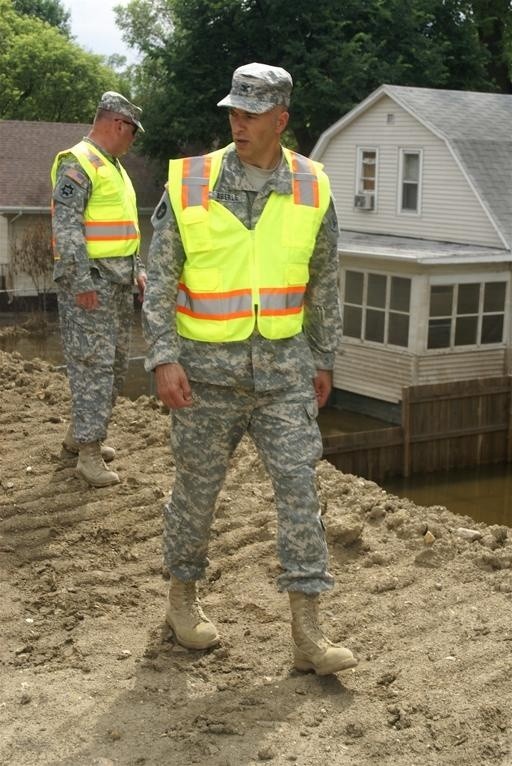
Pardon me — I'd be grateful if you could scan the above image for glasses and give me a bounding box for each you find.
[114,119,139,135]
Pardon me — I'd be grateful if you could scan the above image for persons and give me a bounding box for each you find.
[50,91,147,487]
[141,62,359,675]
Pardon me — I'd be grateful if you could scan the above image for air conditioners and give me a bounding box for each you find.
[354,194,374,210]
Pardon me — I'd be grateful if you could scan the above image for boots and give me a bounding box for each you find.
[73,439,122,488]
[164,564,222,652]
[60,422,117,463]
[287,589,359,678]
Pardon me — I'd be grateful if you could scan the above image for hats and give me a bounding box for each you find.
[96,90,145,134]
[216,62,294,115]
